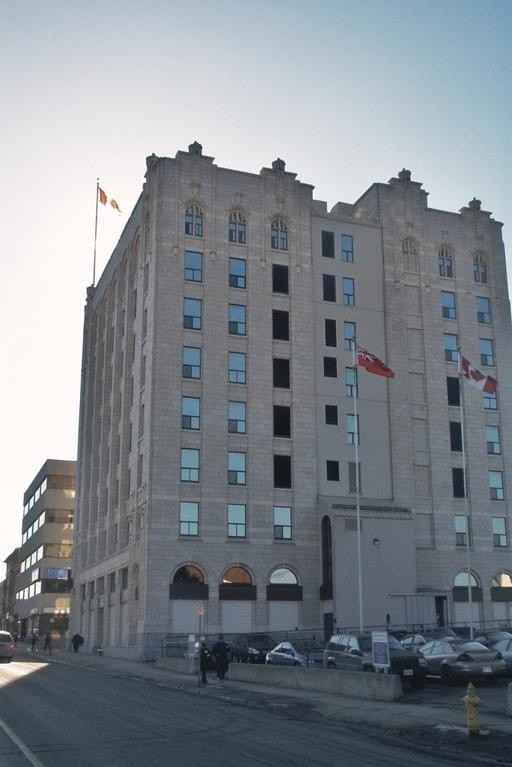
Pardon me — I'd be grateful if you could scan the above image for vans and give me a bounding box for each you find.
[0,629,15,663]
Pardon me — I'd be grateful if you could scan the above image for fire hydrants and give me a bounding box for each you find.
[462,682,483,739]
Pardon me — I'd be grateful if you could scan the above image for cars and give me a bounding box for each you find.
[403,626,512,685]
[183,633,325,668]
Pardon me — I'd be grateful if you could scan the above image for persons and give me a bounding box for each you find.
[212,634,231,681]
[10,629,85,656]
[198,641,212,687]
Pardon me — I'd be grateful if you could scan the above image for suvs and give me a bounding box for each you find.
[323,631,427,690]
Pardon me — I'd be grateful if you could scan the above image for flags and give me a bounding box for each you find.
[457,345,499,400]
[94,176,123,216]
[352,333,395,384]
